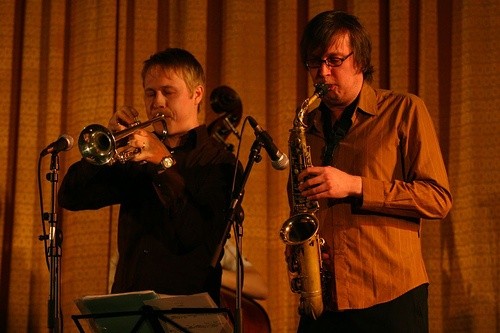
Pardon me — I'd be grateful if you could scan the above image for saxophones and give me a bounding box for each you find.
[280,82,331,320]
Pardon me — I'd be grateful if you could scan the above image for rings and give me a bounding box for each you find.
[142,143,145,148]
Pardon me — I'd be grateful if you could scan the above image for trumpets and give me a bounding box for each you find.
[78,112,169,165]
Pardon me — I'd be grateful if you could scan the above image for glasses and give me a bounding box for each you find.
[303,52,353,68]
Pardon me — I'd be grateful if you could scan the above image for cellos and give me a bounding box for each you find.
[220,286,272,333]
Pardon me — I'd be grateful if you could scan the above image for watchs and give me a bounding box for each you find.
[157,156,173,171]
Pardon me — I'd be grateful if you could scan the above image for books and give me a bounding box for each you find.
[76,290,233,333]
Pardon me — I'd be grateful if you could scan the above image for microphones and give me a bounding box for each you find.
[40,134,74,157]
[247,115,289,171]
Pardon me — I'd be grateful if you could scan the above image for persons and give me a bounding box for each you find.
[284,10,453,333]
[56,47,243,300]
